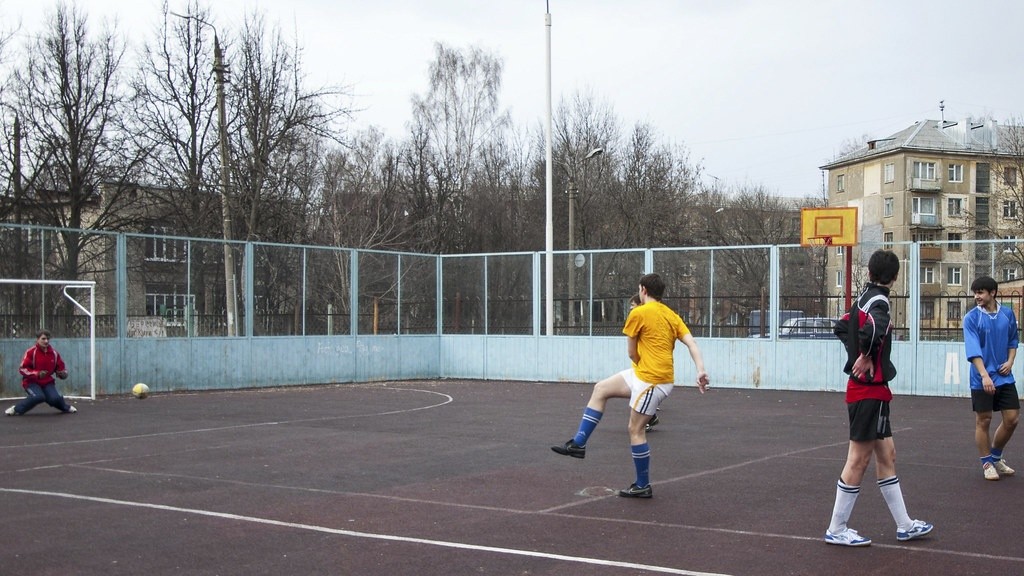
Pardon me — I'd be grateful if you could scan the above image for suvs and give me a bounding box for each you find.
[747,317,842,340]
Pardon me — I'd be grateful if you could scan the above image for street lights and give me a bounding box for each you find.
[169,9,240,337]
[562,146,616,339]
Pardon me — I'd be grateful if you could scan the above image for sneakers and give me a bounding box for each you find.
[5,406,16,415]
[620,481,653,497]
[896,520,934,540]
[995,459,1015,474]
[983,461,999,479]
[825,527,871,545]
[550,439,585,459]
[69,406,77,413]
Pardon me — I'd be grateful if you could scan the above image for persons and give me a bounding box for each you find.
[551,273,710,498]
[962,276,1019,481]
[824,249,934,545]
[4,330,78,415]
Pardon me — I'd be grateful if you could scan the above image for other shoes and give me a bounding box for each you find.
[645,414,658,431]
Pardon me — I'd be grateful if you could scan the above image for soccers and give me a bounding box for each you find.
[132,383,150,399]
